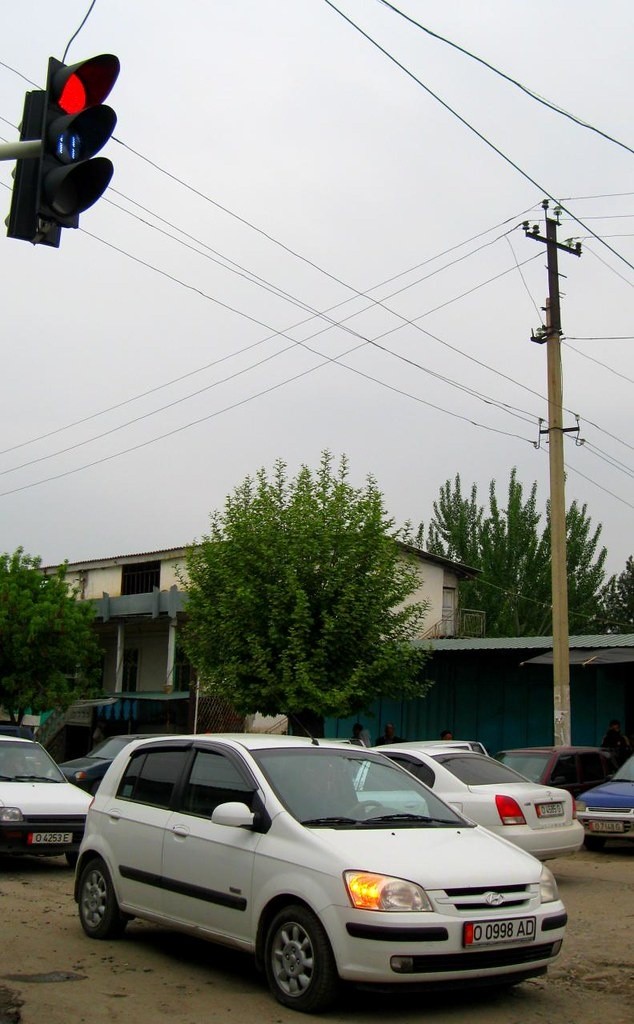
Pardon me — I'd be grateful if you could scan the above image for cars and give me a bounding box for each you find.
[58,733,187,799]
[74,729,570,1013]
[393,739,490,758]
[344,745,586,865]
[574,754,634,854]
[1,732,98,870]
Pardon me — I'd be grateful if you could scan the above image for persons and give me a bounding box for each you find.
[601,719,629,770]
[441,730,452,740]
[352,724,400,748]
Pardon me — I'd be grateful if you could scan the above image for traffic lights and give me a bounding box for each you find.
[39,48,119,231]
